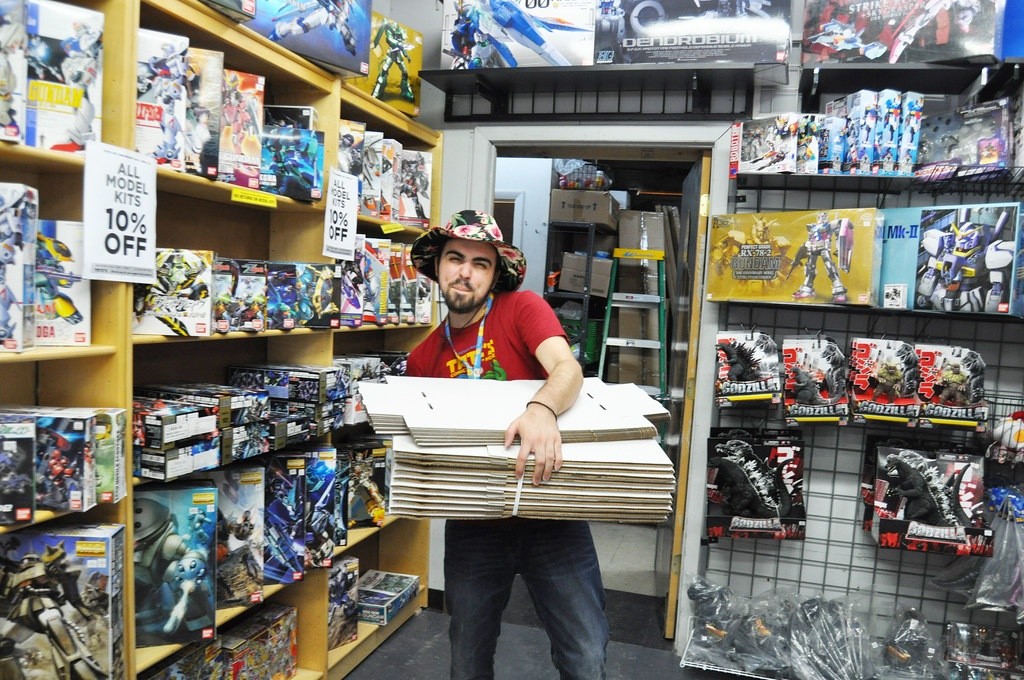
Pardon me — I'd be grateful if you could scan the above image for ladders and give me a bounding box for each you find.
[662,296,689,448]
[597,249,667,452]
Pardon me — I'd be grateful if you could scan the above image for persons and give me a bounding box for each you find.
[406,210,611,680]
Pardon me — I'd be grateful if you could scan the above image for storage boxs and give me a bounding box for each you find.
[1,1,1023,680]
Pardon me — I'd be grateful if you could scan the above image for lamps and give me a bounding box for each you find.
[754,62,788,86]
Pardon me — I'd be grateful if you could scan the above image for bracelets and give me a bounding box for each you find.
[526,401,558,423]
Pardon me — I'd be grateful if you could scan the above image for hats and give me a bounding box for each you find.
[410,209,527,293]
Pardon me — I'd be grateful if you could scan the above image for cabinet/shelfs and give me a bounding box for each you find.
[0,0,444,678]
